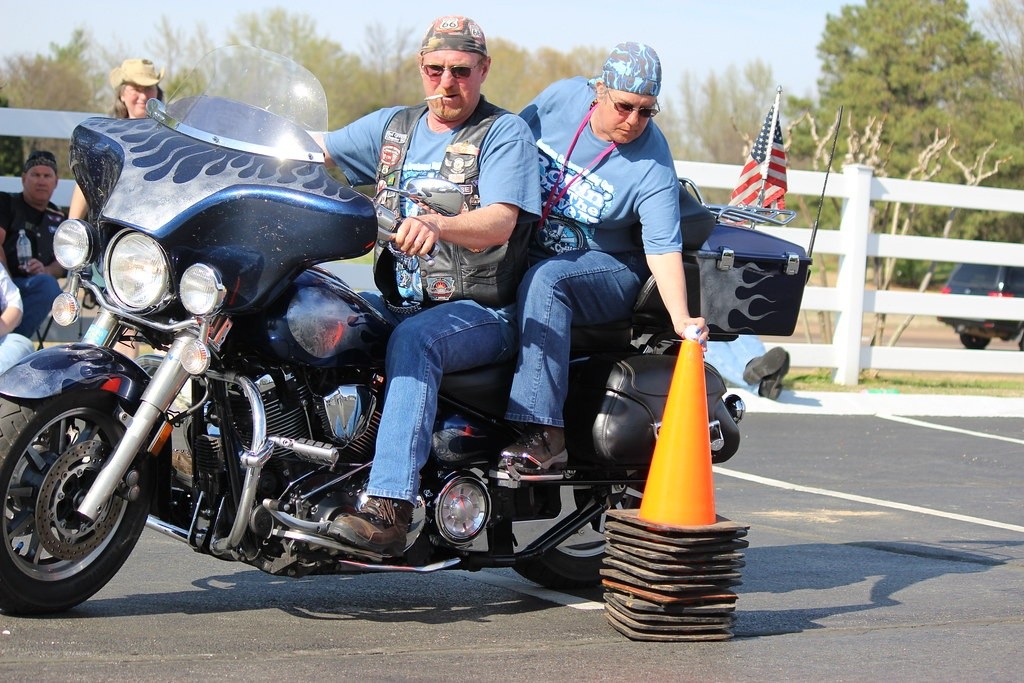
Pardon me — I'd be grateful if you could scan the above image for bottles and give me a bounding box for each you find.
[17,229,32,272]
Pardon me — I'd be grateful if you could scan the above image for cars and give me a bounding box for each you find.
[933,264,1024,351]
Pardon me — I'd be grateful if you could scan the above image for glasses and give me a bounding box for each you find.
[421,57,483,78]
[605,86,661,119]
[25,150,57,164]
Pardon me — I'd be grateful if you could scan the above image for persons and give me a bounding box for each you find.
[0,150,67,340]
[70,57,164,221]
[0,261,36,374]
[304,16,542,554]
[502,43,709,470]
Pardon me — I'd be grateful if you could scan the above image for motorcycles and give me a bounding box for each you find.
[1,42,850,624]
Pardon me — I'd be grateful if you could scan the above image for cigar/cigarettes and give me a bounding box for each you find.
[424,94,444,101]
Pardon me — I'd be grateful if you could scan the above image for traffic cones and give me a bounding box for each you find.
[599,337,754,644]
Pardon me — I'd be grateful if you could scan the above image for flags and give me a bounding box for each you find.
[723,95,789,222]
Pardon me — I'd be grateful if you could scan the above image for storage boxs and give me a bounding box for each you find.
[592,186,814,466]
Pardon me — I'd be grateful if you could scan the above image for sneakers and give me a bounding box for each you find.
[326,497,413,556]
[500,426,570,469]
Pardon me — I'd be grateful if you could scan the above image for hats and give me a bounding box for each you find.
[420,16,488,59]
[603,41,662,96]
[109,58,165,88]
